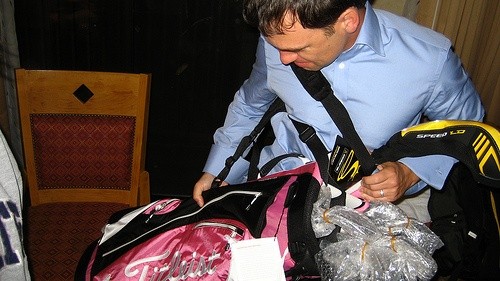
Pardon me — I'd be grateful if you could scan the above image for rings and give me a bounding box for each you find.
[380,189,384,198]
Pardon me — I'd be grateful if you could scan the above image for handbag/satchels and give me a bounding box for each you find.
[77,119,500,281]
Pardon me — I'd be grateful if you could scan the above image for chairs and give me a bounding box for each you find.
[16,69,151,281]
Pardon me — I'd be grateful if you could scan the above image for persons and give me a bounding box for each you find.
[193,0,486,281]
